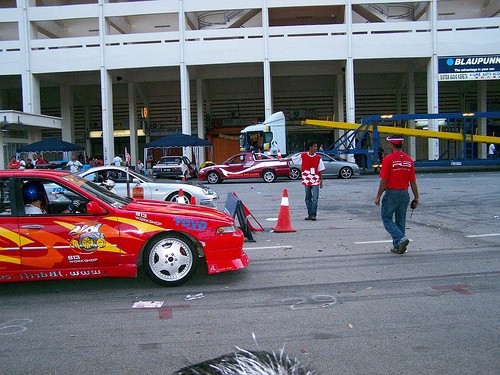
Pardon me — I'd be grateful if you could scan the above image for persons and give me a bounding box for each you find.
[8,154,50,170]
[65,152,83,175]
[136,159,148,178]
[487,139,497,163]
[21,183,80,214]
[373,133,420,255]
[288,139,326,222]
[113,154,123,169]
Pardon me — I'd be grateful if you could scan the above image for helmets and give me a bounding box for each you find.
[21,181,44,203]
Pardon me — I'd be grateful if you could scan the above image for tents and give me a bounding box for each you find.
[143,129,213,180]
[15,137,87,165]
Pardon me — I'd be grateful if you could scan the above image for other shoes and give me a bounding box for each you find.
[311,216,316,220]
[391,248,399,253]
[305,216,311,220]
[399,238,409,254]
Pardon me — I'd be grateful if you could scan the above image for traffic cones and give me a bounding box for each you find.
[273,188,298,233]
[178,188,186,205]
[190,196,196,204]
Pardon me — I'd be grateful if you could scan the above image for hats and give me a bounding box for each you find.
[387,135,404,145]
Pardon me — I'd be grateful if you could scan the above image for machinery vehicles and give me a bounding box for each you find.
[238,111,500,173]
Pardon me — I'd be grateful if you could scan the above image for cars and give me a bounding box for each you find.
[198,152,289,182]
[281,152,362,178]
[43,164,220,214]
[153,157,197,180]
[1,168,252,285]
